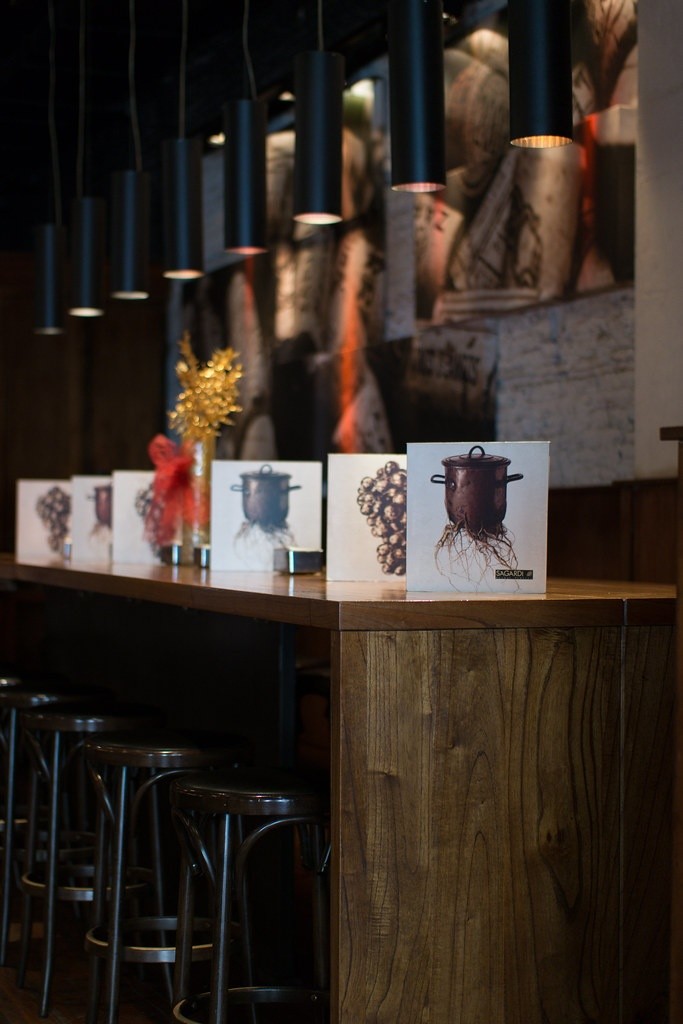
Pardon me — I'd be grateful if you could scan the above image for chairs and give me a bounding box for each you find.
[0,674,331,1024]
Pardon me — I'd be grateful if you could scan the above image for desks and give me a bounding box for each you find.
[0,550,683,1024]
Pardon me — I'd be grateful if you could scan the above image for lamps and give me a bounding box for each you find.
[33,1,574,335]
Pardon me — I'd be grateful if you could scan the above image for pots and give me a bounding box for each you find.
[87,482,111,524]
[230,464,300,529]
[430,446,523,531]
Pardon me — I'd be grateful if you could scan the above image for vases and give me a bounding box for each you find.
[181,431,215,567]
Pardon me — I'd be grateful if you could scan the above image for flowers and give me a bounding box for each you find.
[161,325,246,437]
